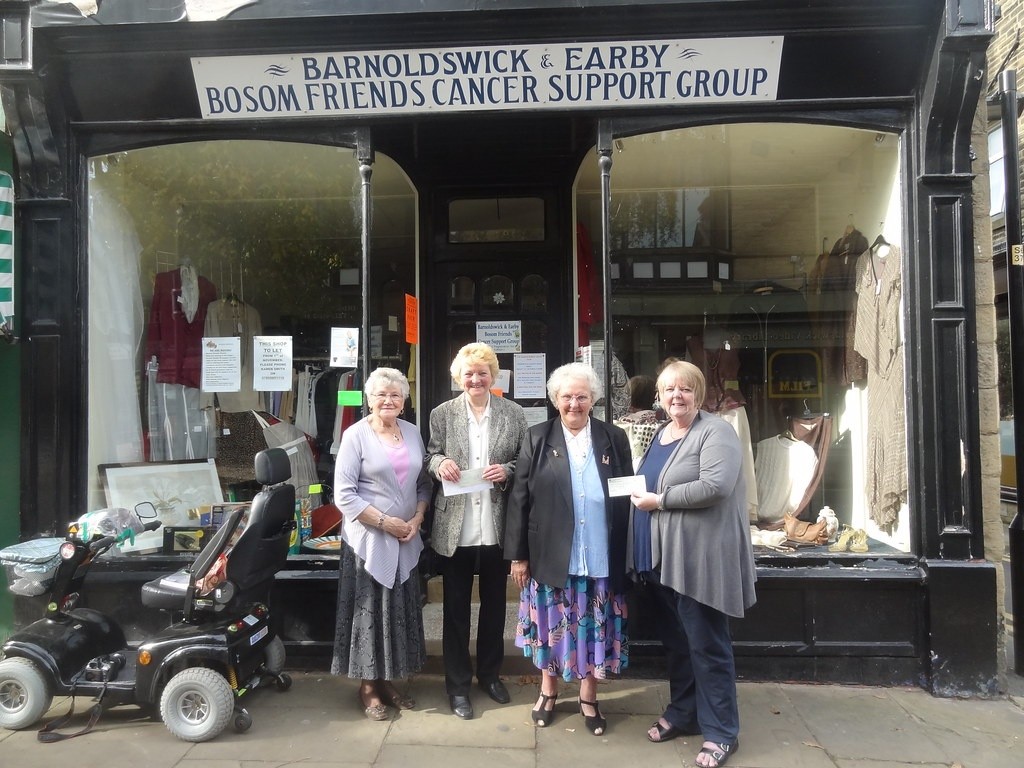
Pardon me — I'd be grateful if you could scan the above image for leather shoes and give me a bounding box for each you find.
[478,678,509,704]
[449,694,473,720]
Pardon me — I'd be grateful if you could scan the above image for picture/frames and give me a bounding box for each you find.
[98,455,226,557]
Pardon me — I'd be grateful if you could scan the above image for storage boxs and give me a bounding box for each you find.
[164,502,302,558]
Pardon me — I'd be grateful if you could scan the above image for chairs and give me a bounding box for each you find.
[141,447,295,608]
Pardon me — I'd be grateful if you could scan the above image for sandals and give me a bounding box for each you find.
[694,738,739,768]
[647,717,683,742]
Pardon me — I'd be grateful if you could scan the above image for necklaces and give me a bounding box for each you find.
[670,425,682,439]
[703,333,722,369]
[387,429,400,441]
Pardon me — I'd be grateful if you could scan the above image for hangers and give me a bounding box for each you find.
[838,214,855,257]
[818,237,828,261]
[292,355,393,376]
[785,399,830,421]
[225,284,242,304]
[870,222,890,250]
[754,274,773,294]
[779,416,797,442]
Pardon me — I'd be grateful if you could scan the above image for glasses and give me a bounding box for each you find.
[371,392,403,401]
[557,393,593,403]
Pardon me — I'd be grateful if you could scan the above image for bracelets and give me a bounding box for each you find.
[511,559,520,563]
[656,495,663,510]
[376,514,387,529]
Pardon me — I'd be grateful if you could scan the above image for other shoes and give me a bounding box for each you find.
[358,687,389,720]
[379,688,417,710]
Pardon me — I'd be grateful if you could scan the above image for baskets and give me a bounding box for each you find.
[0,537,68,599]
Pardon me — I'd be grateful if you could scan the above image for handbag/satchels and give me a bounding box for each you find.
[783,511,828,545]
[610,352,633,420]
[75,507,144,547]
[750,524,795,552]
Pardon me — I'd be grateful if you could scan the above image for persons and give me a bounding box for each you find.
[427,342,529,720]
[625,360,758,768]
[330,367,434,721]
[686,326,746,412]
[503,361,632,736]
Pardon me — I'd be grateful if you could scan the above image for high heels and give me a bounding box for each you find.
[577,696,607,736]
[531,692,559,728]
[828,524,856,552]
[850,528,869,553]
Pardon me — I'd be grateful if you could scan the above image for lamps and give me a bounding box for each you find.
[106,153,118,168]
[615,140,626,153]
[874,133,885,145]
